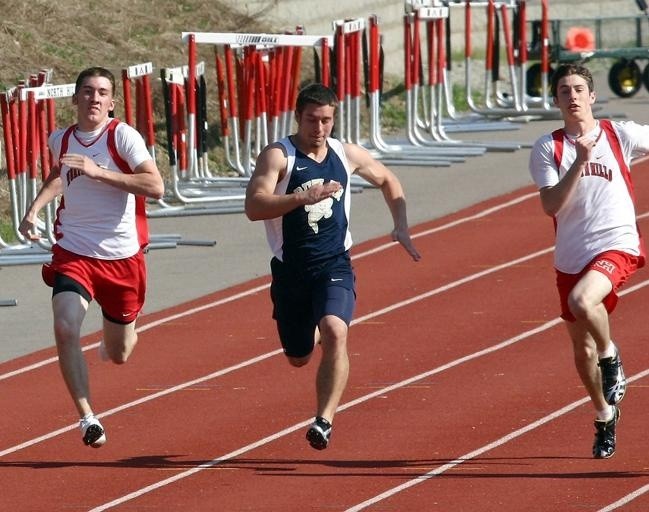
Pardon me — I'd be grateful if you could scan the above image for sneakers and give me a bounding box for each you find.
[592,406,621,459]
[306,416,333,451]
[598,346,626,405]
[80,415,107,448]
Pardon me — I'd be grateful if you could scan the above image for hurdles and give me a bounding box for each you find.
[122,27,377,216]
[333,0,627,168]
[0,69,215,265]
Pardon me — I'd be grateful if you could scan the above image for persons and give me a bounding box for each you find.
[525,63,649,460]
[15,67,163,449]
[246,83,421,449]
